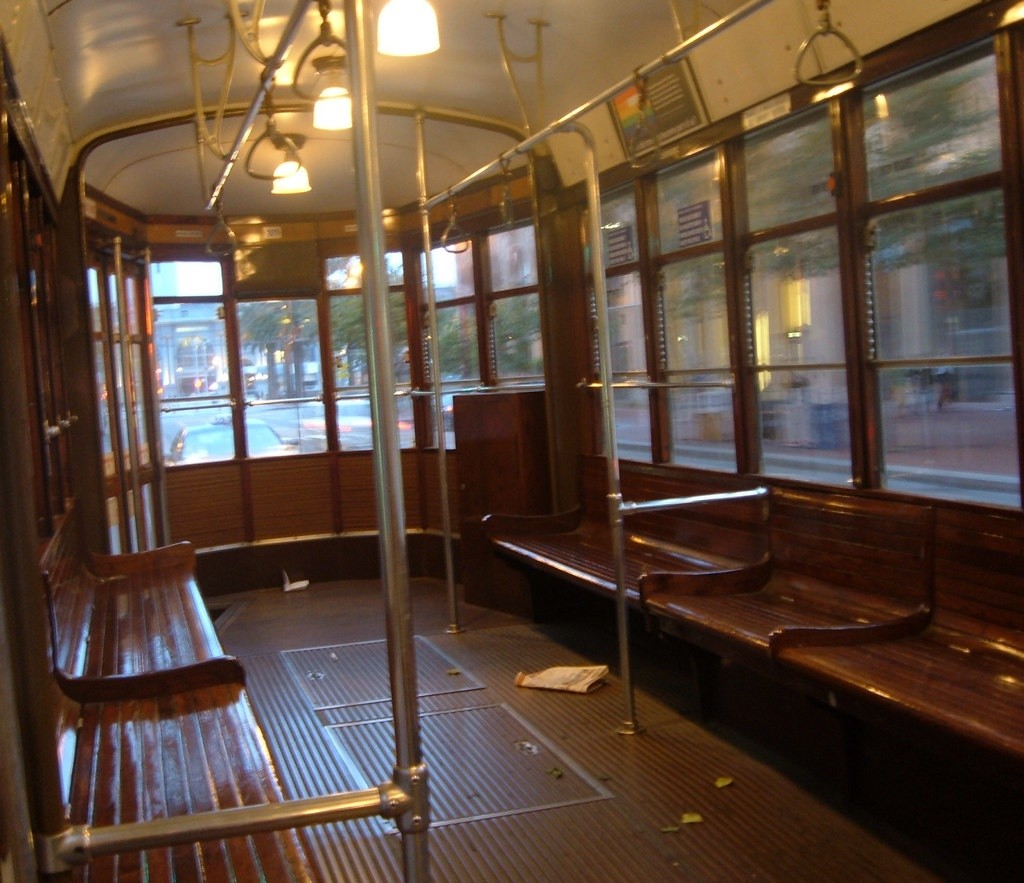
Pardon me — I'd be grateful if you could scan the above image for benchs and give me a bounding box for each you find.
[478,448,1023,809]
[37,501,322,883]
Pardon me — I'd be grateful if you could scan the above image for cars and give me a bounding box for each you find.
[167,414,300,465]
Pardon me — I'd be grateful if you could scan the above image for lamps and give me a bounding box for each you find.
[377,0,440,57]
[270,133,312,194]
[312,55,352,130]
